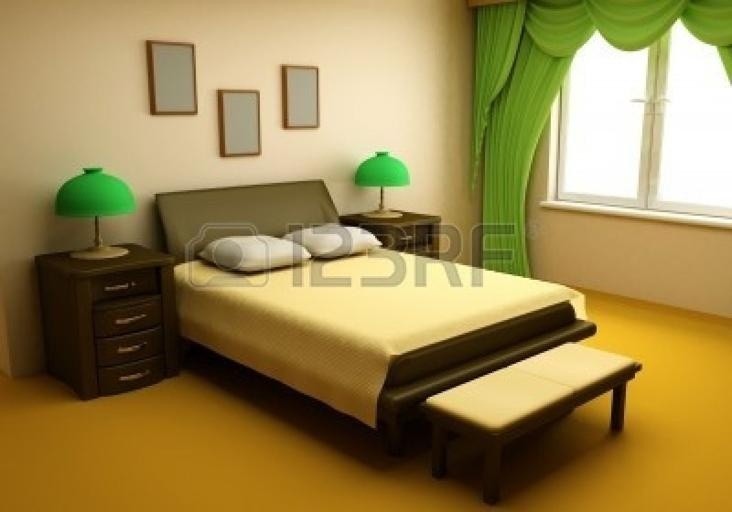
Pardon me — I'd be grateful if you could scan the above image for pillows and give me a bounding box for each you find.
[283,223,382,260]
[198,234,311,273]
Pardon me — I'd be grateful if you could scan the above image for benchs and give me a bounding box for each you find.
[420,342,643,507]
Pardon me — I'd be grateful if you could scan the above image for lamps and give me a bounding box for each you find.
[354,151,410,219]
[55,167,137,260]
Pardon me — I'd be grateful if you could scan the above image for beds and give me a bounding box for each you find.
[154,178,597,458]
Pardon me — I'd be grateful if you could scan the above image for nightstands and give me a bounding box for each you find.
[340,208,442,260]
[33,242,177,401]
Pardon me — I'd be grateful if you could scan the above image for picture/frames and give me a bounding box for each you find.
[146,40,199,115]
[218,89,262,157]
[282,65,320,129]
